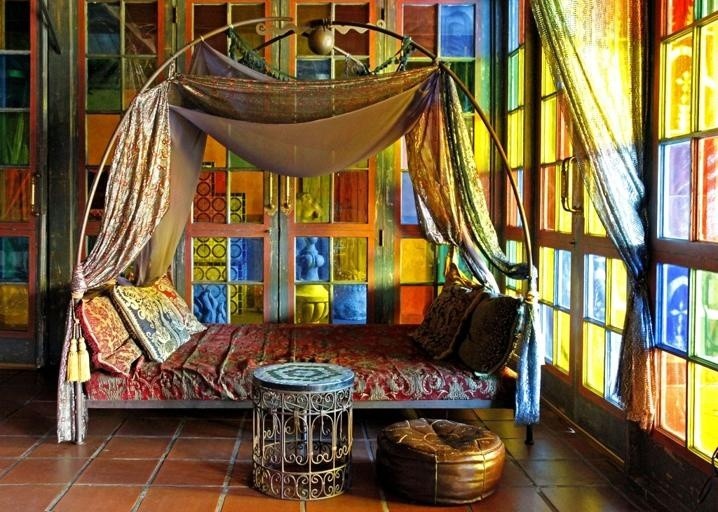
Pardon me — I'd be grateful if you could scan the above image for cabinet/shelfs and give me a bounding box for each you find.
[170,3,389,321]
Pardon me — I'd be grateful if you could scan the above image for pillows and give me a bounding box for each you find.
[154,273,207,334]
[406,264,485,362]
[77,294,141,375]
[112,284,191,362]
[457,285,525,381]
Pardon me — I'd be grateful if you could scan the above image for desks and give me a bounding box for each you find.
[253,361,355,501]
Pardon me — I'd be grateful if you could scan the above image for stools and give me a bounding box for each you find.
[376,418,505,503]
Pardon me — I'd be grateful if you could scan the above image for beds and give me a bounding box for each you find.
[75,268,538,446]
